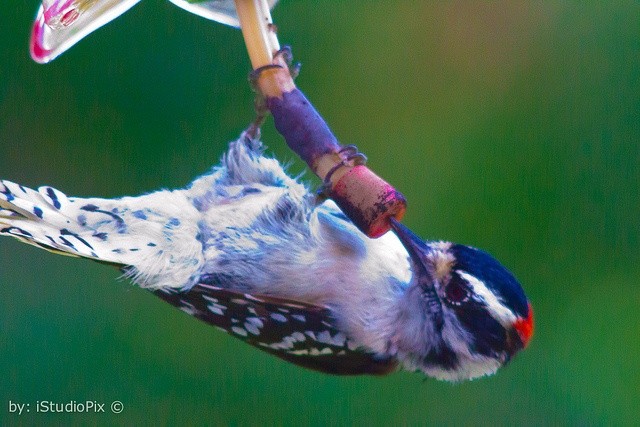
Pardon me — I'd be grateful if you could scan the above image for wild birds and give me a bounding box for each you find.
[0,45,534,382]
